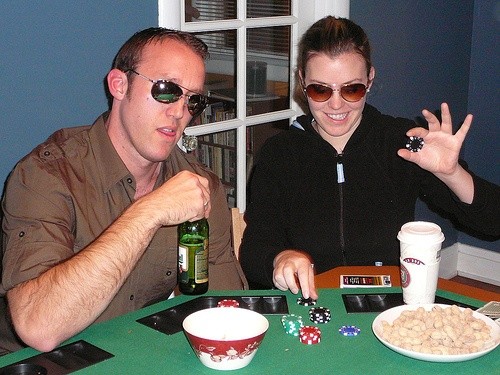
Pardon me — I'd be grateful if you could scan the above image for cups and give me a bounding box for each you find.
[397,220,445,304]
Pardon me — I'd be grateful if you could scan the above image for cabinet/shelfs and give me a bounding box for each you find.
[185,74,288,210]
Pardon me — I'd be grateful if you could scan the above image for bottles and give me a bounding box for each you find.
[177,217,209,296]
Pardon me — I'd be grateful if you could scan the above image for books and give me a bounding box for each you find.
[182,87,280,182]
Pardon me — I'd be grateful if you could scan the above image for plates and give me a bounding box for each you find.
[372,303,500,363]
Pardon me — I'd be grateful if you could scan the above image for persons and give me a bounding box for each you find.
[238,16,500,300]
[0,28,243,356]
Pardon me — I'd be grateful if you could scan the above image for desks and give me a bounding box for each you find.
[0,265,500,375]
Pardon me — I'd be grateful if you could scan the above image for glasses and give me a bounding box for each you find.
[123,68,210,117]
[299,78,369,102]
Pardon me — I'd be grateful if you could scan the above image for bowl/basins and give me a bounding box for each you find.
[182,307,269,370]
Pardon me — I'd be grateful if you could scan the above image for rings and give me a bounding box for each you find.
[204,202,208,206]
